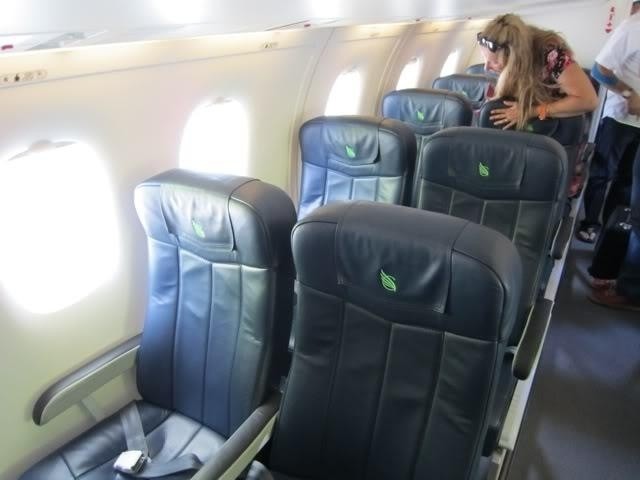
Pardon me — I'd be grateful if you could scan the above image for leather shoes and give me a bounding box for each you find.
[591,286,640,312]
[592,278,617,289]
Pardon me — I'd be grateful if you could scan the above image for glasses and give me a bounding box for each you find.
[477,32,508,53]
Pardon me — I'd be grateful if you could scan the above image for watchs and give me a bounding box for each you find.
[622,85,633,99]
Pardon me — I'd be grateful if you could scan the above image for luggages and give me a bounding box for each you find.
[592,204,632,278]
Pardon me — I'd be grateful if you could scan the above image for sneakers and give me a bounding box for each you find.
[575,226,598,244]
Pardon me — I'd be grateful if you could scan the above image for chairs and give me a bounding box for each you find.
[298,64,609,298]
[211,199,553,479]
[17,168,300,478]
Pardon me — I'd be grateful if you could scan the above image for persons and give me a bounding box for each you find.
[575,10,640,244]
[587,144,639,312]
[475,12,601,130]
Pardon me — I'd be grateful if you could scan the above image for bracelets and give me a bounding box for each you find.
[538,101,549,121]
